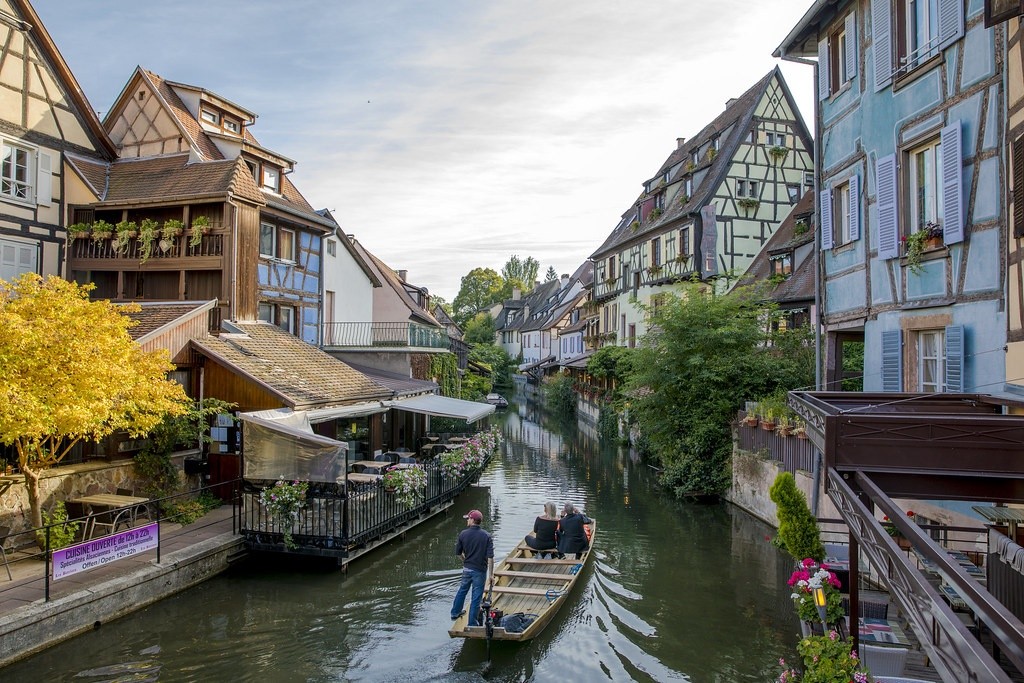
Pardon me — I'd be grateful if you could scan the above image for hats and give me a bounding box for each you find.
[463,510,483,519]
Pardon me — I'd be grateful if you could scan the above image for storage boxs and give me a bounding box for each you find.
[184,456,203,475]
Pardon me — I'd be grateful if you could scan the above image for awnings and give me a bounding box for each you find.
[519,350,589,373]
[392,393,497,424]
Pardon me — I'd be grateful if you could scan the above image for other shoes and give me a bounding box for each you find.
[451,609,466,620]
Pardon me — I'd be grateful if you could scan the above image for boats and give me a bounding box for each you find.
[486,392,508,408]
[449,512,596,644]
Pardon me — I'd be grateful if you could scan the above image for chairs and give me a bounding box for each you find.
[63,487,152,543]
[0,526,13,581]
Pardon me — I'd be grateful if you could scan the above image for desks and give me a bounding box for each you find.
[889,546,988,667]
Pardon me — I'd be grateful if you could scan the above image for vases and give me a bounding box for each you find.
[813,621,830,637]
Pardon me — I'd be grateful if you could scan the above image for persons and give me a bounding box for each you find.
[451,509,494,626]
[524,502,591,553]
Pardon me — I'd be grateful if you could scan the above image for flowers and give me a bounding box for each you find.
[902,236,907,241]
[883,515,896,536]
[774,656,818,683]
[830,631,867,683]
[787,557,842,605]
[259,424,505,527]
[906,511,914,518]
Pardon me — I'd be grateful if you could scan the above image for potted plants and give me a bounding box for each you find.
[158,219,185,252]
[908,220,943,274]
[112,219,138,254]
[67,222,92,247]
[137,218,160,264]
[89,220,114,248]
[188,216,211,248]
[566,145,812,441]
